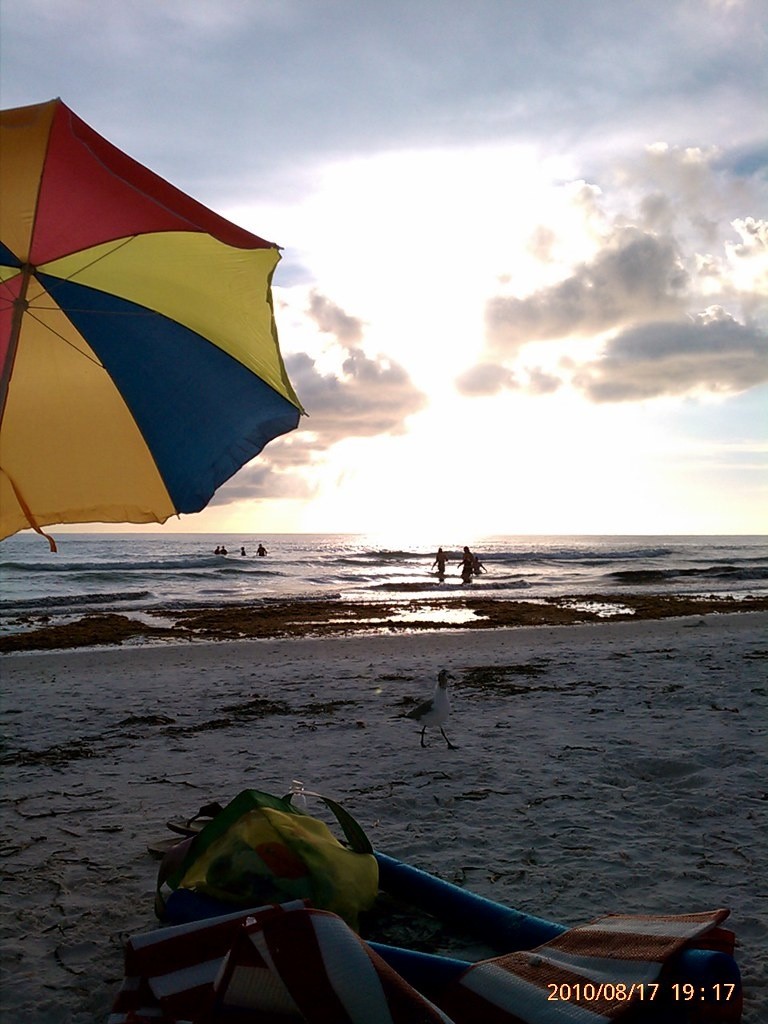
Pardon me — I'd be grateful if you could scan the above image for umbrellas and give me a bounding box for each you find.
[1,96,314,547]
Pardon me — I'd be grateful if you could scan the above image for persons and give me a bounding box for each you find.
[241,547,245,556]
[214,546,227,555]
[433,548,448,575]
[256,544,267,556]
[458,546,487,584]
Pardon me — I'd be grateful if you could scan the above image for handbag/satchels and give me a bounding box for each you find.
[154,790,381,940]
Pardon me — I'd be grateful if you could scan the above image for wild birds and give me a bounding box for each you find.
[392,669,462,750]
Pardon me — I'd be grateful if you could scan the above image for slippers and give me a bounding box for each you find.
[146,801,226,859]
